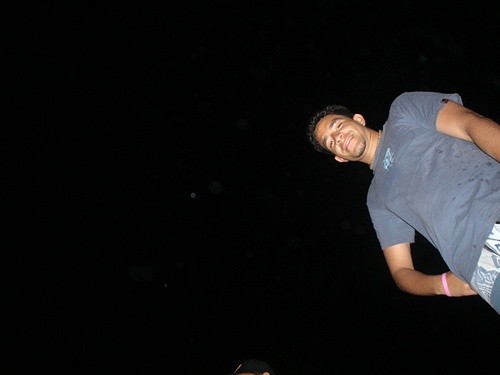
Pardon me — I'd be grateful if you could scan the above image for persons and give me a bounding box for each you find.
[307,91,500,316]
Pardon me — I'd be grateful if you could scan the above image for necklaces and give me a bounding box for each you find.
[367,129,380,172]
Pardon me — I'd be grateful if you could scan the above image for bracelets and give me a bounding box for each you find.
[441,272,451,300]
